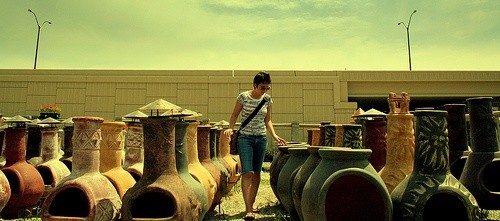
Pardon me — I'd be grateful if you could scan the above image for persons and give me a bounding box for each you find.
[224,72,288,220]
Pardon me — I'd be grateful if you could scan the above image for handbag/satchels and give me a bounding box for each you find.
[228,129,239,155]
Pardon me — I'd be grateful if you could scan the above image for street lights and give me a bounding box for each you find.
[398,10,417,70]
[28,7,52,70]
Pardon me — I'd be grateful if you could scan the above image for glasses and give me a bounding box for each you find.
[260,86,271,91]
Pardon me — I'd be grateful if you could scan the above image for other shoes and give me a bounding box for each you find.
[252,207,260,213]
[244,212,255,221]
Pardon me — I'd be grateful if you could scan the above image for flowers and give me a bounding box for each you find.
[39,103,57,113]
